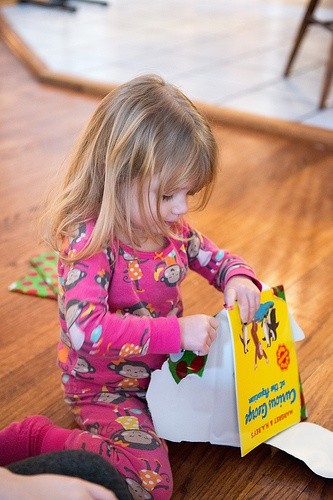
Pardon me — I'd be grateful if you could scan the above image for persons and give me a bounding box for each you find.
[0,449,139,500]
[0,72,262,500]
